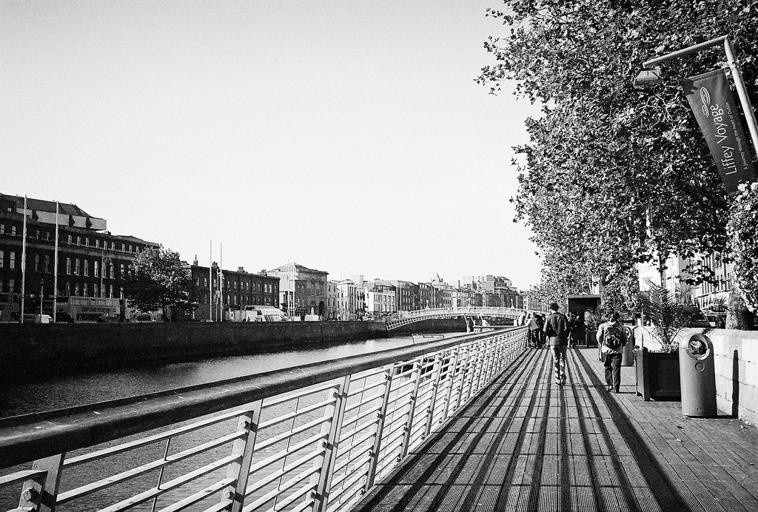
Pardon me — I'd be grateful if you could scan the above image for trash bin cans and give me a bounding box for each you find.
[621,326,633,366]
[677,333,717,418]
[0,311,2,319]
[104,313,108,321]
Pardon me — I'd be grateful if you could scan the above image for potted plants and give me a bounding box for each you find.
[631,280,700,402]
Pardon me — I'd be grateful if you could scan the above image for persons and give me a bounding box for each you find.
[596,312,628,393]
[543,303,571,385]
[526,312,586,348]
